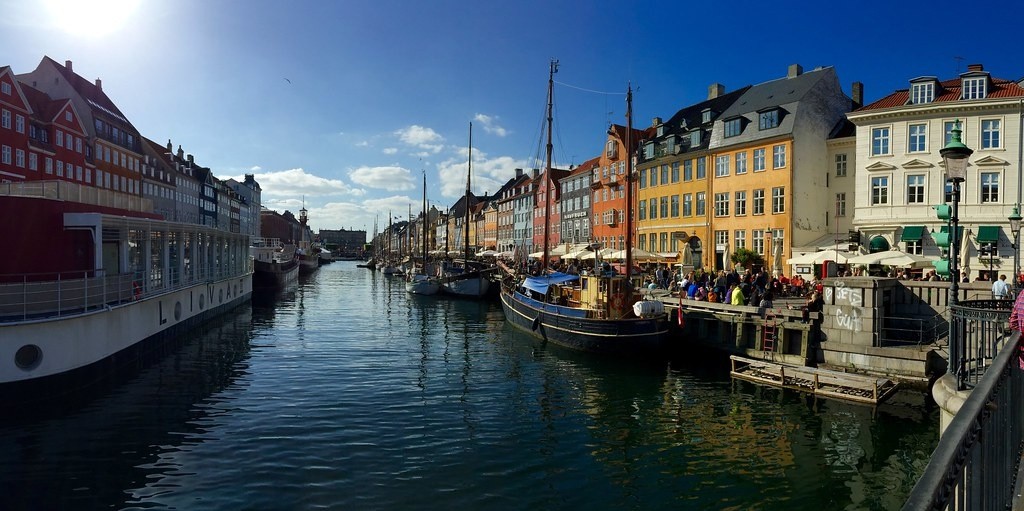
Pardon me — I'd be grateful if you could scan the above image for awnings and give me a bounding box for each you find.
[940,226,963,240]
[977,225,1000,243]
[901,226,924,242]
[869,236,888,250]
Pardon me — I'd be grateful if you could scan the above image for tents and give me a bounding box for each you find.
[430,250,514,256]
[529,249,662,260]
[786,250,932,268]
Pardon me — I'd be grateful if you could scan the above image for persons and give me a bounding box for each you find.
[431,254,617,277]
[962,272,968,283]
[897,270,907,278]
[976,273,989,281]
[680,267,823,323]
[837,268,861,277]
[992,274,1009,320]
[909,270,940,281]
[631,264,678,291]
[1009,271,1024,371]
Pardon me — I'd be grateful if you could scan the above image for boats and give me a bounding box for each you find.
[298,248,319,273]
[250,238,301,284]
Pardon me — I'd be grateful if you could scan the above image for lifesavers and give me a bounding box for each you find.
[611,293,626,310]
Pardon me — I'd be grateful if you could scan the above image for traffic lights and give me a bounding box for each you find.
[931,204,952,278]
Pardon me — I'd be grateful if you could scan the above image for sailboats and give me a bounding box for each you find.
[500,60,669,351]
[376,204,408,274]
[437,122,490,297]
[405,173,440,295]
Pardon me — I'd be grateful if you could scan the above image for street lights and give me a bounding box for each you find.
[766,227,772,269]
[977,243,998,281]
[939,118,974,303]
[590,240,602,269]
[1008,208,1023,299]
